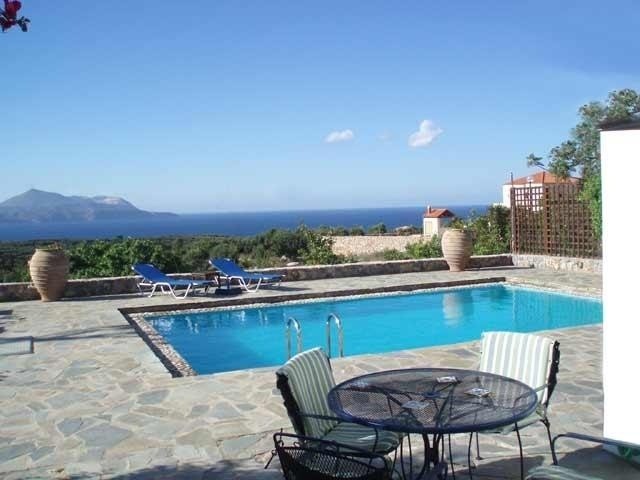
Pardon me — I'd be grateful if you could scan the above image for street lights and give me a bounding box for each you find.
[527,176,534,211]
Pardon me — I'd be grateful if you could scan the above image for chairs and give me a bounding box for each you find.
[131,264,209,299]
[210,258,286,294]
[274,434,388,480]
[264,348,412,480]
[468,332,559,480]
[528,435,640,480]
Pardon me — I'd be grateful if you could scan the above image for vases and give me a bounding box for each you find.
[441,229,474,271]
[29,247,68,301]
